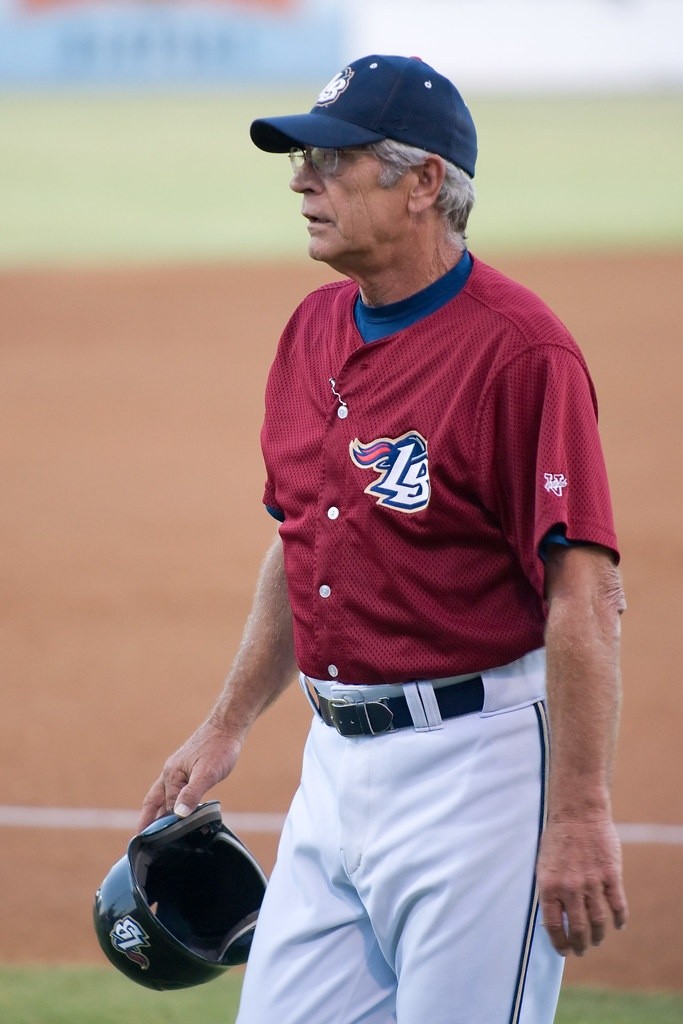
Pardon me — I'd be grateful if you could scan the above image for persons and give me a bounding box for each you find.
[131,55,629,1023]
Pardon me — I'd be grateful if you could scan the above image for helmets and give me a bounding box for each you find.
[90,800,268,992]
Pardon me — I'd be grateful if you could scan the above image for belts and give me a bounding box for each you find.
[314,674,485,737]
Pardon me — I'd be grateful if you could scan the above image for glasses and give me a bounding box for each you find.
[288,139,399,175]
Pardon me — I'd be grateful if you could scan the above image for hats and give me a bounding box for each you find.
[250,54,478,179]
